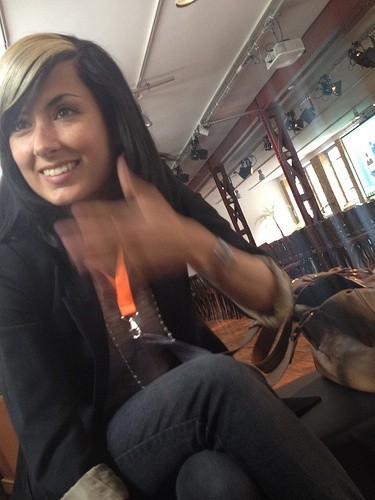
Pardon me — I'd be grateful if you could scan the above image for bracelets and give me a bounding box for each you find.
[202,238,235,286]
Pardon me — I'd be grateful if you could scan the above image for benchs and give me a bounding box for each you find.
[274,371,375,500]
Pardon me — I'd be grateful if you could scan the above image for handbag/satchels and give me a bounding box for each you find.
[294,266,375,393]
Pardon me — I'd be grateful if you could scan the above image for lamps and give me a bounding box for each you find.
[262,132,283,151]
[233,167,254,179]
[353,109,362,120]
[235,190,241,199]
[347,48,365,69]
[137,101,153,128]
[316,75,342,97]
[173,166,189,183]
[257,168,265,181]
[285,111,304,132]
[299,97,316,123]
[190,143,208,160]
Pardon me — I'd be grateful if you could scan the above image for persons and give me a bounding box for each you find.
[0,32,356,500]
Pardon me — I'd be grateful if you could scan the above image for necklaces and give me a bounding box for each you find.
[85,245,174,388]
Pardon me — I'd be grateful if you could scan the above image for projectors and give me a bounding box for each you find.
[265,38,305,70]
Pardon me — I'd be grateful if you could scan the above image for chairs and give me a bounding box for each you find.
[188,197,375,322]
